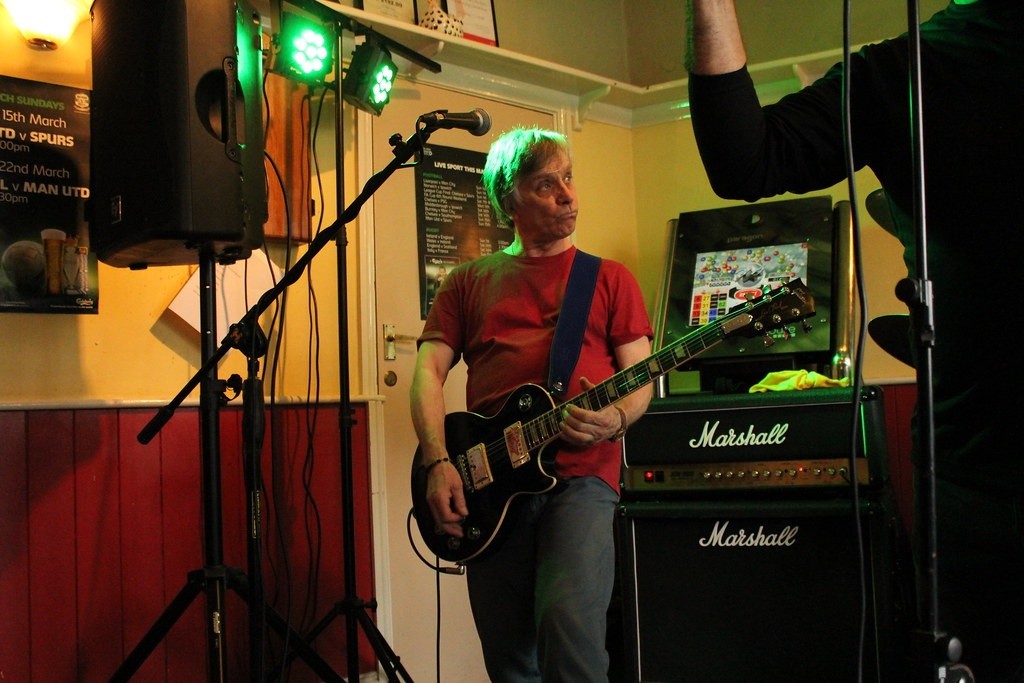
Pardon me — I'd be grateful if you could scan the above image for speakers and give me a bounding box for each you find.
[602,488,896,683]
[88,0,269,270]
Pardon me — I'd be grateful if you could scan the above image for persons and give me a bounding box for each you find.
[408,126,656,683]
[682,0,1024,683]
[425,265,449,305]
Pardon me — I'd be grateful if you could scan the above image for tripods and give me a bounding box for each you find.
[268,23,414,683]
[107,265,347,683]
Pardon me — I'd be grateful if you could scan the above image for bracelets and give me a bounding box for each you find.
[420,457,454,472]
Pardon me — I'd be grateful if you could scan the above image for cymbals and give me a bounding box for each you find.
[868,313,917,369]
[865,188,898,237]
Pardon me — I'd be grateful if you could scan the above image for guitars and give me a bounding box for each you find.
[411,276,818,567]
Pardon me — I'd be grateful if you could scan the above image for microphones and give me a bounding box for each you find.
[418,107,492,136]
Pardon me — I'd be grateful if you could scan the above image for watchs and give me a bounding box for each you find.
[609,407,627,445]
